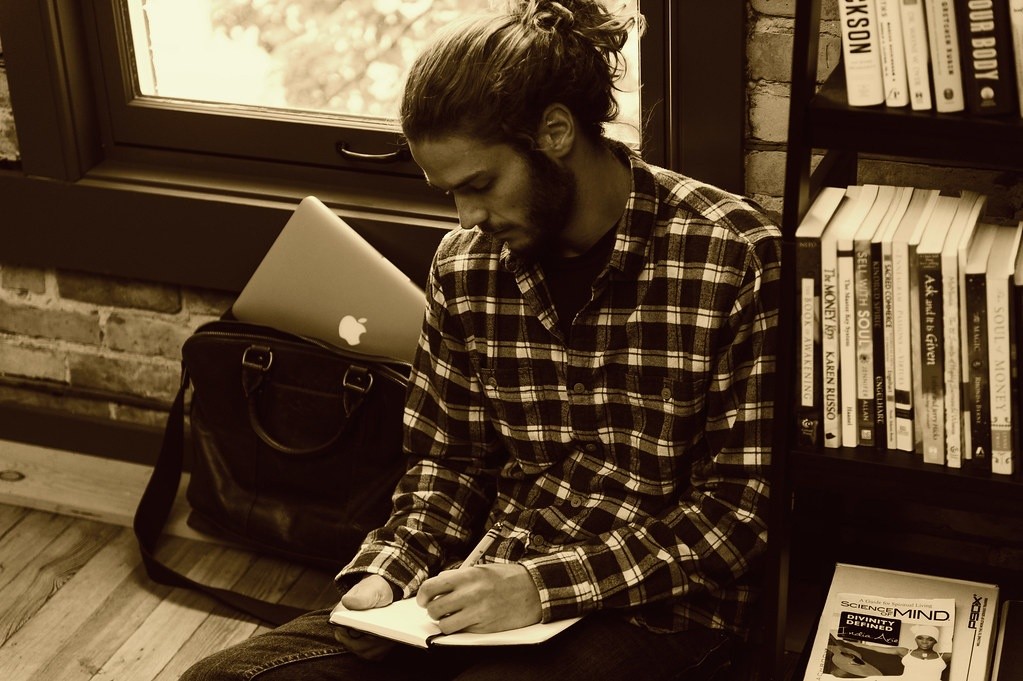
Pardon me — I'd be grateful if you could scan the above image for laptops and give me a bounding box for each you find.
[231,195,428,362]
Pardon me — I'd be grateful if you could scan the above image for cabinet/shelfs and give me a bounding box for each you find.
[770,0,1023,677]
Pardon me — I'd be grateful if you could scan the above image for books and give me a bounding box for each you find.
[793,0,1022,679]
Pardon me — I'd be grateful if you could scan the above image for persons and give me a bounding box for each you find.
[175,0,787,681]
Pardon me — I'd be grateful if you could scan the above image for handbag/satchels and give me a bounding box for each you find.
[133,323,411,626]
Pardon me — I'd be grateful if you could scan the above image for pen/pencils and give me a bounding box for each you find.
[456,520,506,570]
